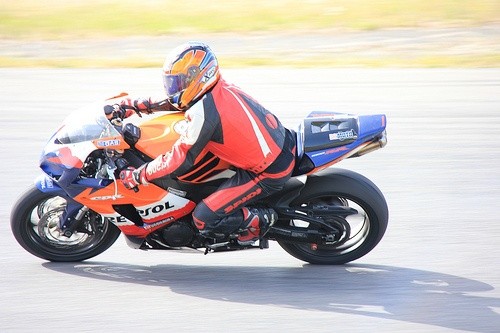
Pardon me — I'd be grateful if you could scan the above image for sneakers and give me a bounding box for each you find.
[237,207,275,245]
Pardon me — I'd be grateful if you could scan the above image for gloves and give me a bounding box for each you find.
[121,98,151,118]
[120,162,150,190]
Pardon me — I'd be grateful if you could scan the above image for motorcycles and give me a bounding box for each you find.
[10,91,388,265]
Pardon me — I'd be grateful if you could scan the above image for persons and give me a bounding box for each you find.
[118,40,299,246]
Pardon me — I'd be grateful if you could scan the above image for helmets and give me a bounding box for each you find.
[163,43,219,110]
